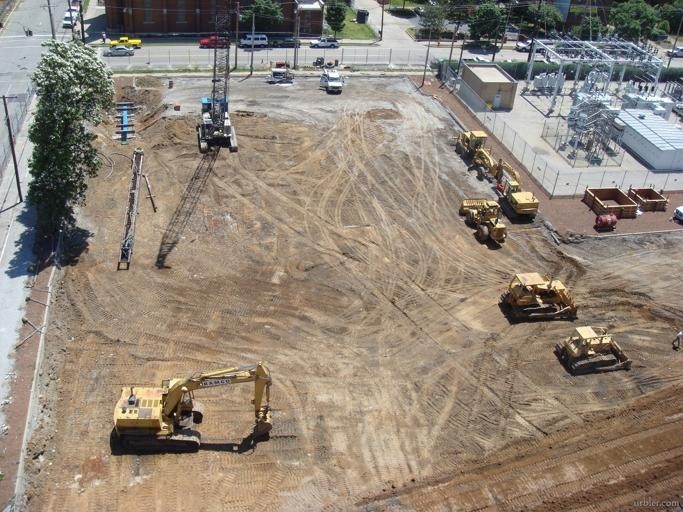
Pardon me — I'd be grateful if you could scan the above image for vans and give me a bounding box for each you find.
[239,33,268,47]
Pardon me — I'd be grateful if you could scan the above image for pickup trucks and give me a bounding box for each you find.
[200,34,229,48]
[109,35,143,49]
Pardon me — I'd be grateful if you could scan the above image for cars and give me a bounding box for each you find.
[667,46,682,57]
[273,38,300,47]
[413,5,427,15]
[428,0,439,5]
[515,38,545,53]
[102,45,135,56]
[673,205,682,221]
[310,37,339,47]
[61,0,82,28]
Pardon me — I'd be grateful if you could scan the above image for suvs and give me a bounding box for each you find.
[319,70,345,94]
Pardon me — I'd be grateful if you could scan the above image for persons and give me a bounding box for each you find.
[101,31,106,44]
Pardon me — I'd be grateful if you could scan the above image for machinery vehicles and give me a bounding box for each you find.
[451,128,496,178]
[501,270,579,322]
[459,196,507,243]
[112,361,275,453]
[493,154,539,221]
[194,8,238,154]
[554,325,631,375]
